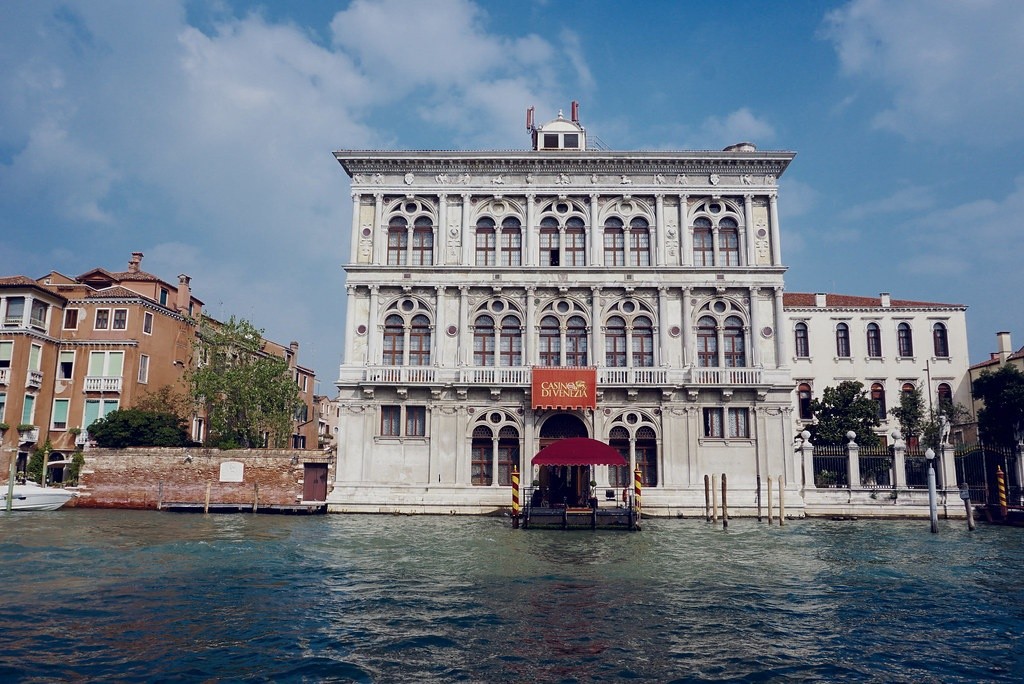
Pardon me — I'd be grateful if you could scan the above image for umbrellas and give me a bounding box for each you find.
[532,437,628,506]
